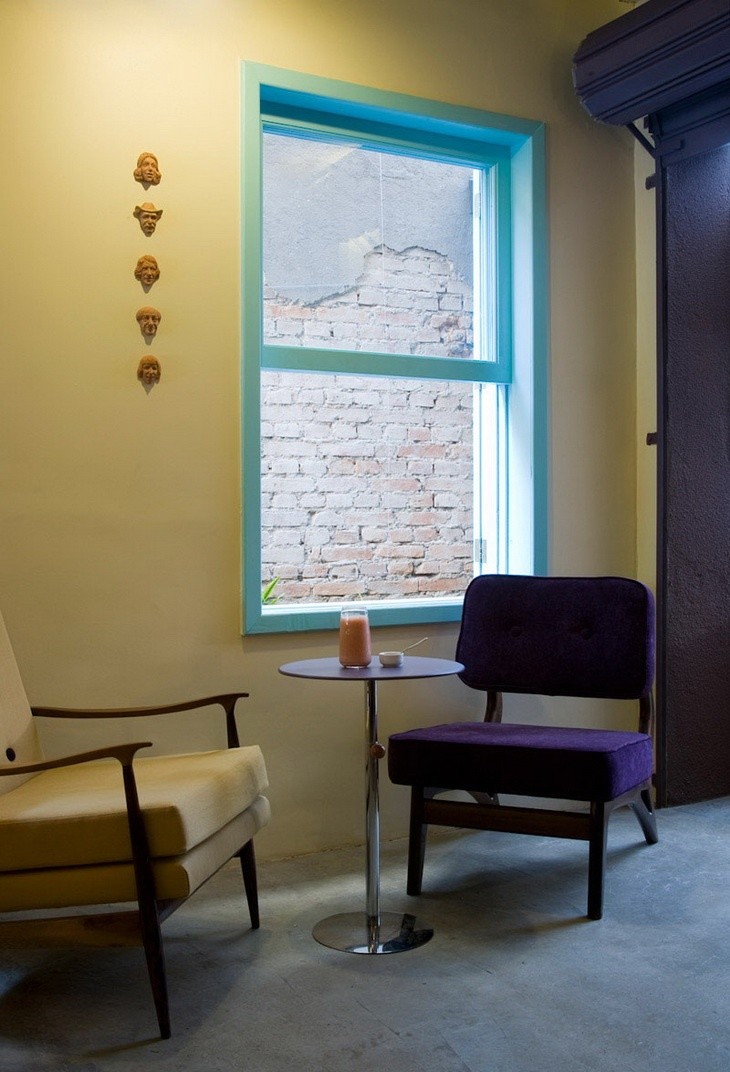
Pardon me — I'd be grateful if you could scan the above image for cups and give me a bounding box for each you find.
[339,605,372,668]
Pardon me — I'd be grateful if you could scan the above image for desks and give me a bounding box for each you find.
[278,656,466,955]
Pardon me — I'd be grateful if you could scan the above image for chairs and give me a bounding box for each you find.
[388,574,658,921]
[0,614,270,1038]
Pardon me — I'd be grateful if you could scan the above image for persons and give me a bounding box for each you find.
[133,202,164,237]
[135,307,162,336]
[136,355,162,385]
[133,151,163,185]
[134,255,159,286]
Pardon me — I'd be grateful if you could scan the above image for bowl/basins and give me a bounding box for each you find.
[378,652,403,667]
